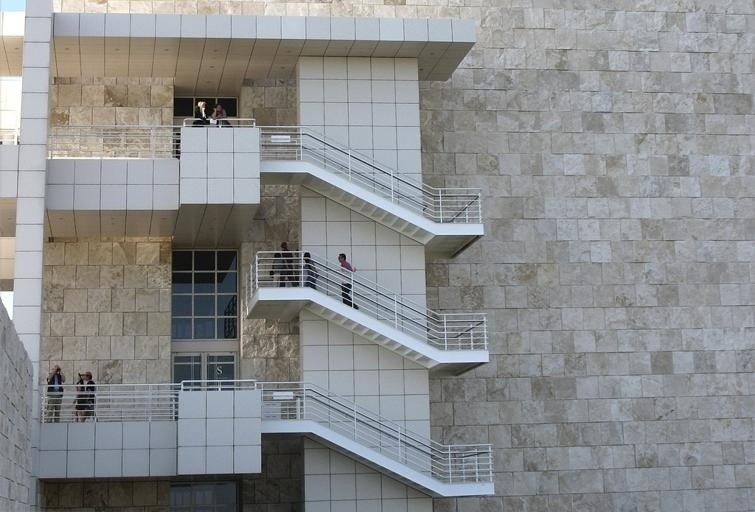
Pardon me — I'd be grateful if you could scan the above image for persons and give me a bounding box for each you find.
[337,253,359,310]
[211,103,232,127]
[191,101,211,127]
[269,241,321,291]
[44,365,98,422]
